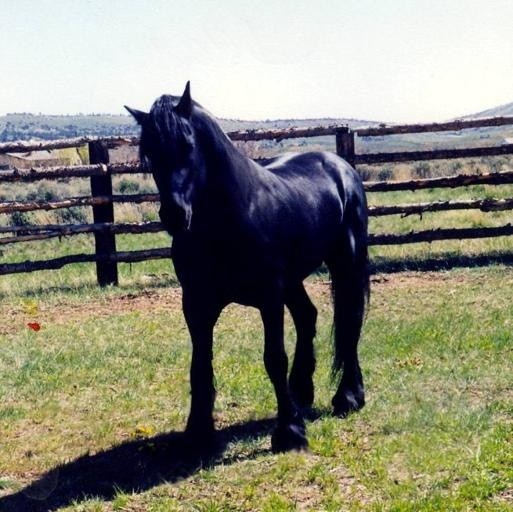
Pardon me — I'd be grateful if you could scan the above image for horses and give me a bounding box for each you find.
[122,79,372,477]
